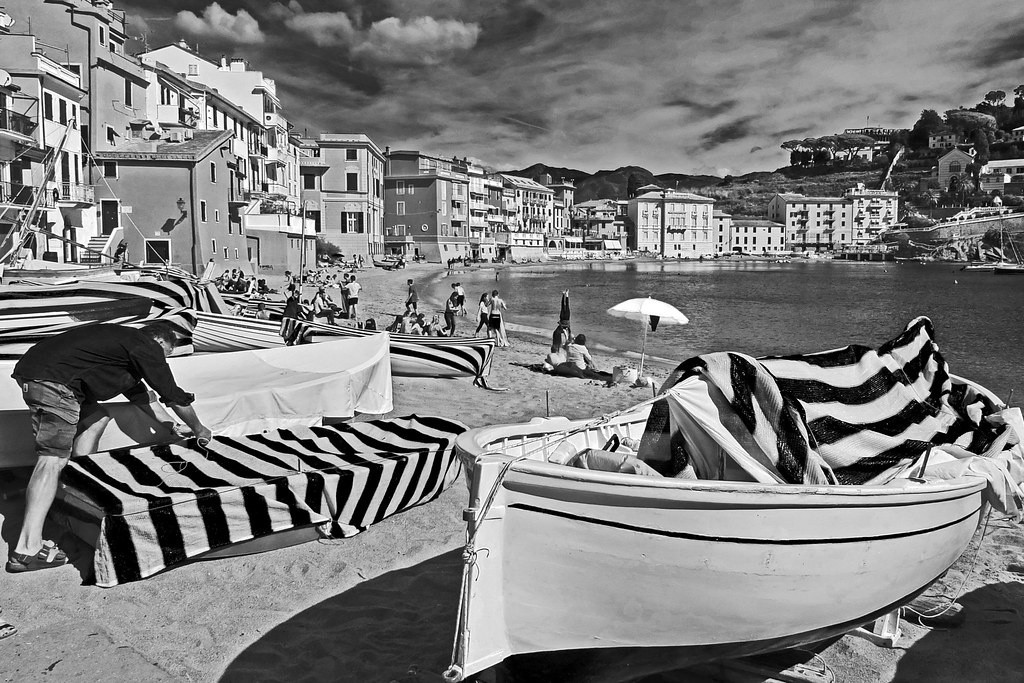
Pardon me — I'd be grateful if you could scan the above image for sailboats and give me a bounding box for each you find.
[994,206,1024,273]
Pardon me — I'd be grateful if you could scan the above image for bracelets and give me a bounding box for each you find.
[170,423,180,435]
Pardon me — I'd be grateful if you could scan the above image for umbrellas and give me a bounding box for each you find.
[607,295,689,376]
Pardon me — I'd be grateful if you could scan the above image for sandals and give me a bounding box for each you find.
[0,604,18,639]
[5,544,69,573]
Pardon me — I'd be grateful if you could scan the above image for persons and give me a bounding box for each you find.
[452,257,455,268]
[443,292,459,336]
[405,279,419,316]
[474,293,489,338]
[5,322,211,573]
[215,265,342,326]
[447,259,450,269]
[485,290,507,347]
[339,273,361,322]
[551,289,572,353]
[458,255,461,267]
[386,310,448,337]
[353,253,364,269]
[452,283,468,317]
[568,334,600,372]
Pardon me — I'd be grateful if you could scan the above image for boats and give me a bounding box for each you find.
[281,313,500,378]
[439,311,1014,683]
[962,261,995,272]
[192,301,285,352]
[0,115,216,362]
[52,411,470,552]
